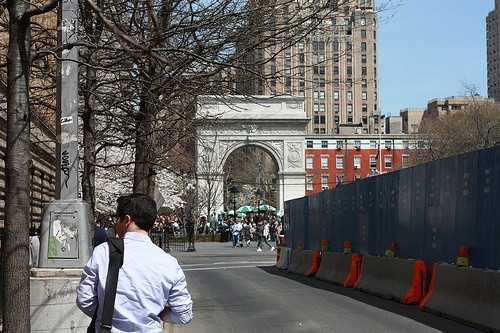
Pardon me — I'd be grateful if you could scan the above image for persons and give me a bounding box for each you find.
[76,193,193,333]
[28,226,40,267]
[154,215,182,235]
[95,216,117,246]
[198,214,283,251]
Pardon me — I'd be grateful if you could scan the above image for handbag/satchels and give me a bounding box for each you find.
[87,319,95,333]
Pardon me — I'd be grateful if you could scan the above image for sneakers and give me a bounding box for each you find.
[257,248,262,251]
[270,246,275,252]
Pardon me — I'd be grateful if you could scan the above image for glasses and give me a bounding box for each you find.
[111,214,124,224]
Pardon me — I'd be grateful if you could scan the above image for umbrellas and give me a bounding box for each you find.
[214,205,284,217]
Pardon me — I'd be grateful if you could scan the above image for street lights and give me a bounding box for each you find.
[230,186,239,219]
[254,188,261,216]
